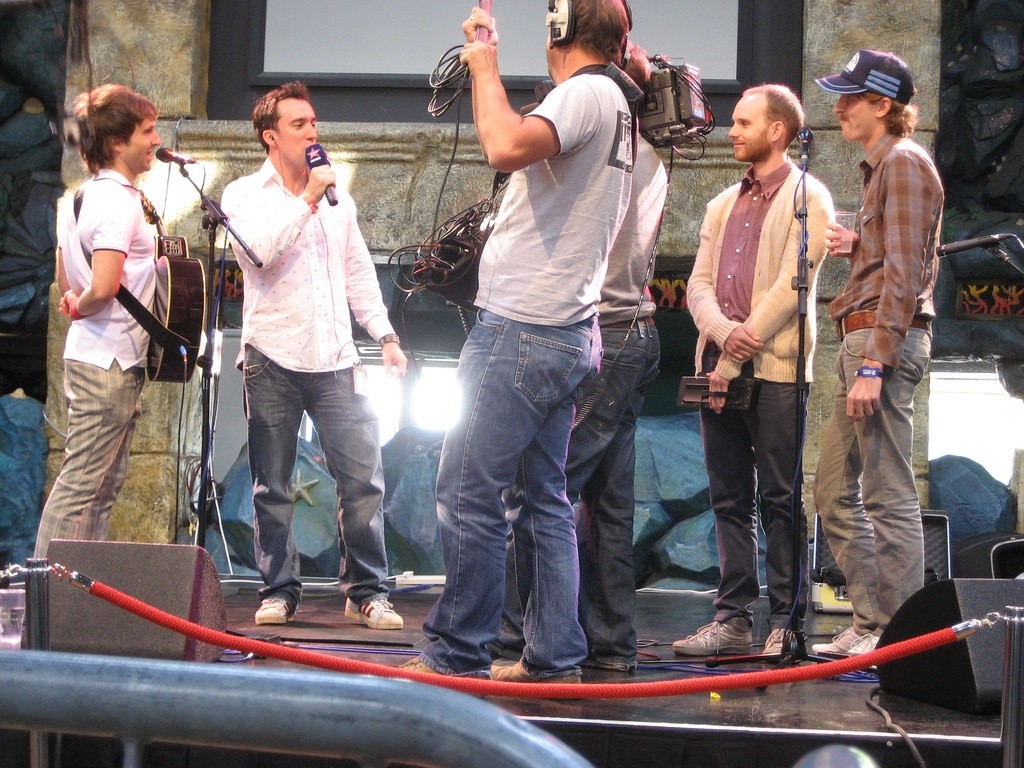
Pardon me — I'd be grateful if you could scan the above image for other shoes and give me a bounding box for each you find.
[582,659,626,670]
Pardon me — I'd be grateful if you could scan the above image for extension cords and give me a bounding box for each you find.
[396,574,448,587]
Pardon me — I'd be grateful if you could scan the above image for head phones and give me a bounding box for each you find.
[546,0,575,50]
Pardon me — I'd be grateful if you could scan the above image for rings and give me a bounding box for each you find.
[470,16,473,20]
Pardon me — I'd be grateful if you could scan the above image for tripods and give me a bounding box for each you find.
[703,167,878,691]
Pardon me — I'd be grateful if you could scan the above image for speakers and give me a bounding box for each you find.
[811,510,1024,718]
[22,537,228,664]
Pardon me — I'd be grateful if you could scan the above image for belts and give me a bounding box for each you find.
[837,310,930,341]
[601,316,655,328]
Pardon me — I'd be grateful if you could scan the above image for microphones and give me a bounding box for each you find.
[305,144,338,206]
[155,148,195,164]
[797,127,813,168]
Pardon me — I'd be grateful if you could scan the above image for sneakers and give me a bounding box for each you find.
[255,598,294,625]
[847,634,880,657]
[491,665,581,683]
[812,626,861,654]
[672,621,753,655]
[762,628,786,656]
[345,597,403,629]
[397,657,441,674]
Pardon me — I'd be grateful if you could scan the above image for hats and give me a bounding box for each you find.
[815,49,913,104]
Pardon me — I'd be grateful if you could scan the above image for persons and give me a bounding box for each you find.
[34,82,161,568]
[670,82,835,662]
[219,81,408,630]
[395,0,668,688]
[814,49,945,668]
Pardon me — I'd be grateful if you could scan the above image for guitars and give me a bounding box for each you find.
[140,188,207,385]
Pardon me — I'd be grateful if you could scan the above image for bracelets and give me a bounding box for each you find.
[70,299,84,320]
[303,196,318,214]
[854,366,883,380]
[380,333,400,346]
